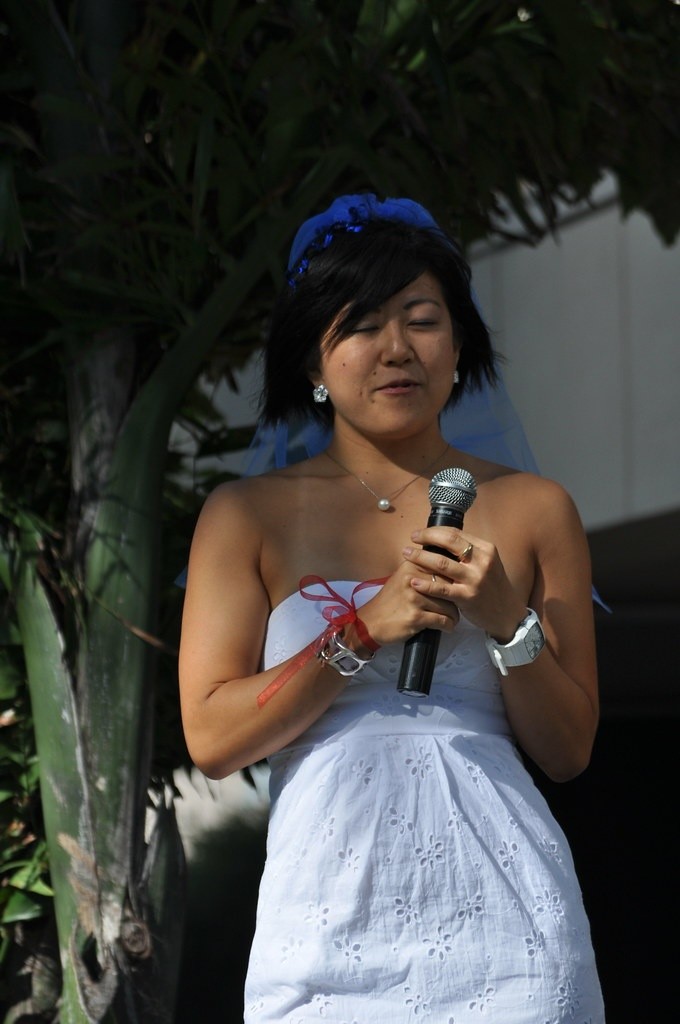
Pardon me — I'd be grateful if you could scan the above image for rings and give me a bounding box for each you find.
[458,542,473,561]
[431,573,436,582]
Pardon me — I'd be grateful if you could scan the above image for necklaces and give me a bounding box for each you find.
[324,443,451,512]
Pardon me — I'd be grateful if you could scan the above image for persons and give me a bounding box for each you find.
[179,196,605,1024]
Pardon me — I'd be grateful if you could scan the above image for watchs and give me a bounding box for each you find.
[485,607,547,676]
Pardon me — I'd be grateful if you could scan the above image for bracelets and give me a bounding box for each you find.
[317,621,376,676]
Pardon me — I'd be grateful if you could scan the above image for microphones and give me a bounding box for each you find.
[394,465,478,698]
